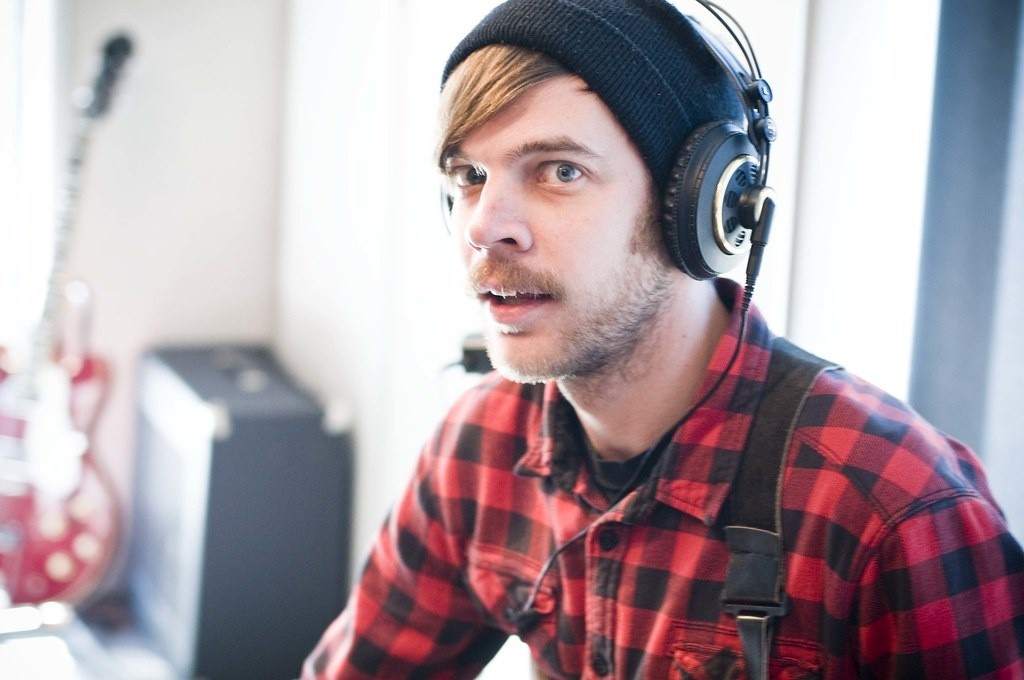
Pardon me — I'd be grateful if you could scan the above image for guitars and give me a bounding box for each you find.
[0,25,140,624]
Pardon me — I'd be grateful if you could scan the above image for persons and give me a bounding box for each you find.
[301,0,1024,680]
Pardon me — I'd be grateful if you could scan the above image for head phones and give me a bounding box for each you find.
[663,0,777,281]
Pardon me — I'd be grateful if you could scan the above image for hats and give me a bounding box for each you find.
[440,0,746,214]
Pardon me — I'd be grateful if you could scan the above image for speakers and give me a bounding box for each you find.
[123,343,358,680]
